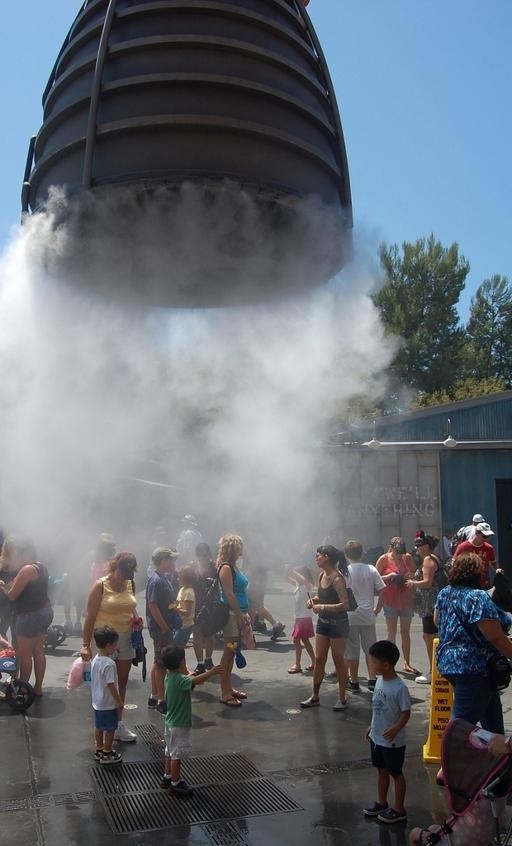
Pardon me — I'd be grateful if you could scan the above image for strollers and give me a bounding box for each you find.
[406,716,512,846]
[40,572,67,649]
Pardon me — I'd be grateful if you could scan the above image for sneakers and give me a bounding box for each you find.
[167,781,194,797]
[101,749,123,764]
[160,778,170,788]
[347,682,361,692]
[415,672,434,685]
[368,679,377,693]
[95,748,104,761]
[147,698,158,709]
[192,664,206,674]
[157,701,169,715]
[114,723,137,742]
[204,658,216,669]
[270,623,286,640]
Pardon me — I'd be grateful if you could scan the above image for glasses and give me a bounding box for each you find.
[479,535,490,540]
[414,543,427,547]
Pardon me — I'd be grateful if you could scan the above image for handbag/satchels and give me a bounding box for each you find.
[490,652,511,692]
[192,599,230,637]
[345,587,358,611]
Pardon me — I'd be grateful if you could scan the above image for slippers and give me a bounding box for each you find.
[402,666,421,675]
[35,693,44,698]
[287,665,302,674]
[233,690,247,700]
[218,696,241,708]
[305,664,314,671]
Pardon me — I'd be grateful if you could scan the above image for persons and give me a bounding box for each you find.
[0,530,285,796]
[284,513,511,844]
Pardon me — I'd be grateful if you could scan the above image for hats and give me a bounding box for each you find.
[476,524,493,536]
[151,545,180,562]
[390,536,406,555]
[471,514,486,523]
[119,558,137,581]
[180,514,198,527]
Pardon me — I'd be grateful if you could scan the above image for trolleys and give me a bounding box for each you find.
[0,647,34,712]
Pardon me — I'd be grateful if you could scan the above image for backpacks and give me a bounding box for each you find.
[435,563,451,586]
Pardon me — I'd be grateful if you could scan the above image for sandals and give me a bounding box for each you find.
[299,698,321,708]
[378,807,408,823]
[332,701,350,710]
[361,800,389,816]
